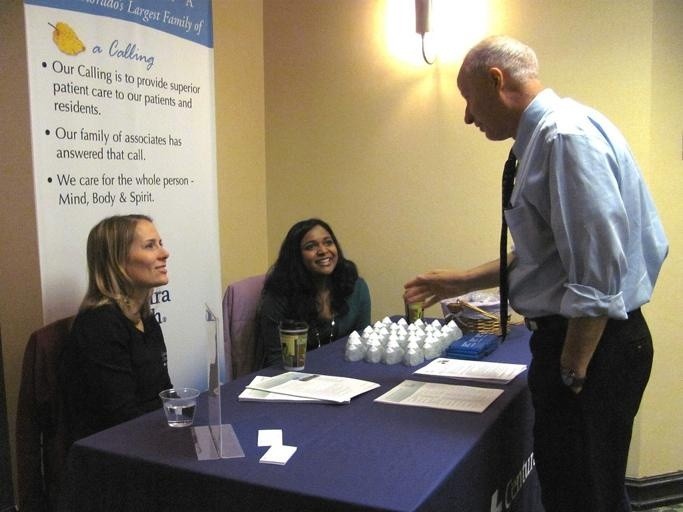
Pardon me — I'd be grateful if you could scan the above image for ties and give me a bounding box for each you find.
[499,151,517,343]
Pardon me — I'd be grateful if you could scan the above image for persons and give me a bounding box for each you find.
[401,32,671,512]
[59,213,178,438]
[248,216,372,374]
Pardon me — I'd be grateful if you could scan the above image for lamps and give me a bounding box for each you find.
[415,0,437,66]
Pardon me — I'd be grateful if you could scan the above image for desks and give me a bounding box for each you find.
[72,316,533,512]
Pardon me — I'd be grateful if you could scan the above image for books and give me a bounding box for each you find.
[237,375,351,405]
[245,370,382,403]
[412,355,529,383]
[373,378,504,414]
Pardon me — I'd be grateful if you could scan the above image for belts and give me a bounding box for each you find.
[524,315,569,332]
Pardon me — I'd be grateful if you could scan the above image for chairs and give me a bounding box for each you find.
[20,315,77,510]
[225,272,268,380]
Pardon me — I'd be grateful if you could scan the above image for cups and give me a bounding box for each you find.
[403,300,425,324]
[278,319,309,371]
[159,388,201,428]
